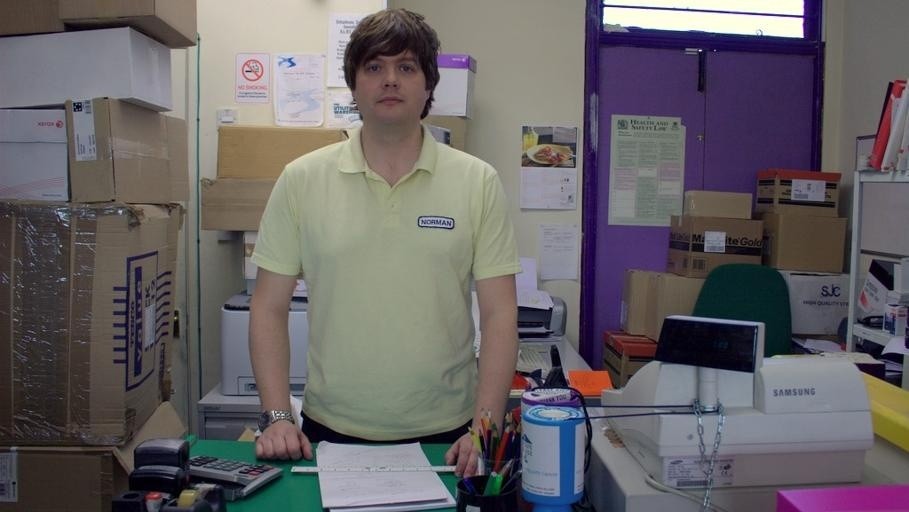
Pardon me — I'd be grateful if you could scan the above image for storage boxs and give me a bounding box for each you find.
[420,52,478,153]
[0,0,199,512]
[603,167,853,389]
[200,124,347,301]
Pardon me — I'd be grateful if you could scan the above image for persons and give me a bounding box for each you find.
[248,8,523,479]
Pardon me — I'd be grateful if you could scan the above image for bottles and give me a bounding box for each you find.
[523,126,538,152]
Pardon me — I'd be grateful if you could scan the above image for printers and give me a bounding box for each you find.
[517,296,567,339]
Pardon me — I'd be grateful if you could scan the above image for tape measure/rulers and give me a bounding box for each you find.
[291,465,457,472]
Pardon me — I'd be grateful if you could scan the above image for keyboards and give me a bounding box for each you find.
[516,343,550,379]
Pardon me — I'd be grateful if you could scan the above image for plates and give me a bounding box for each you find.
[527,143,574,165]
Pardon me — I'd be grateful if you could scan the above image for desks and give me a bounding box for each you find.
[176,433,594,511]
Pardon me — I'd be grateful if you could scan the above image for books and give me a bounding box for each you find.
[870,79,909,173]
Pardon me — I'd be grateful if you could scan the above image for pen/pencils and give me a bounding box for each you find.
[461,408,522,496]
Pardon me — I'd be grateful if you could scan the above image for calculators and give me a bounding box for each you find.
[188,455,283,496]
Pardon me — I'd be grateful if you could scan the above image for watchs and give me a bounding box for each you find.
[256,407,294,432]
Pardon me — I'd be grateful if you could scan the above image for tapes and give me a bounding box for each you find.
[177,490,202,509]
